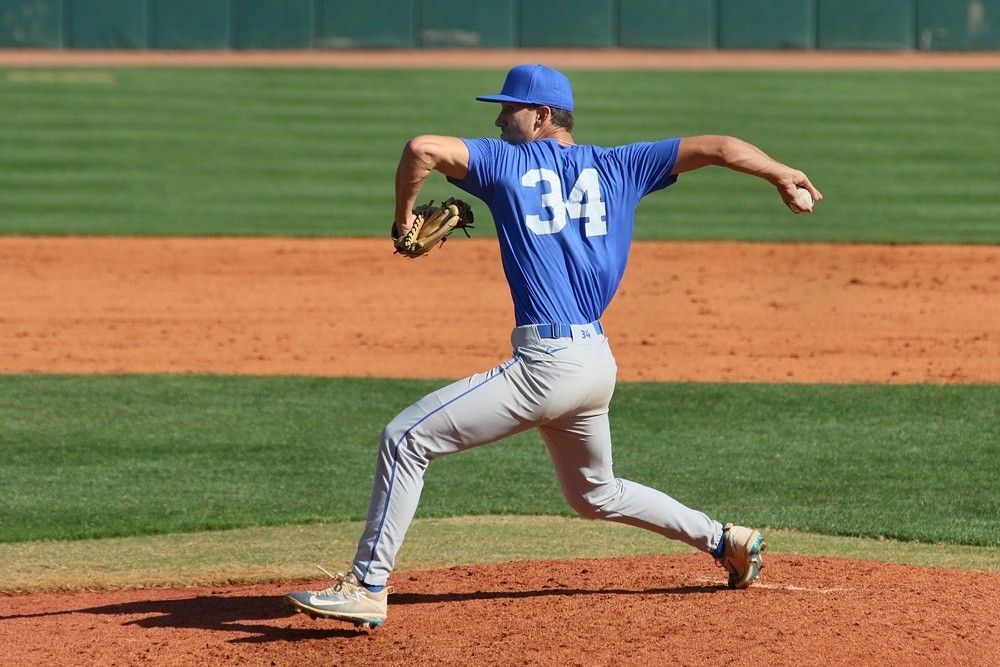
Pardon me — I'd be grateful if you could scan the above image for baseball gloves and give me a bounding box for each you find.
[390,195,474,259]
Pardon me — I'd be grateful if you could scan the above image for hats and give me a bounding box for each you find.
[475,64,574,112]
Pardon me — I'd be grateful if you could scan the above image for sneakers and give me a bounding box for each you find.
[715,523,766,589]
[287,562,394,630]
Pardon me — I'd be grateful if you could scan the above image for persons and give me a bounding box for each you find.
[283,64,823,629]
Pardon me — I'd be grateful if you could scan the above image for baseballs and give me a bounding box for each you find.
[796,188,815,209]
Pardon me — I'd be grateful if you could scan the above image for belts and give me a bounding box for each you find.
[537,321,602,338]
